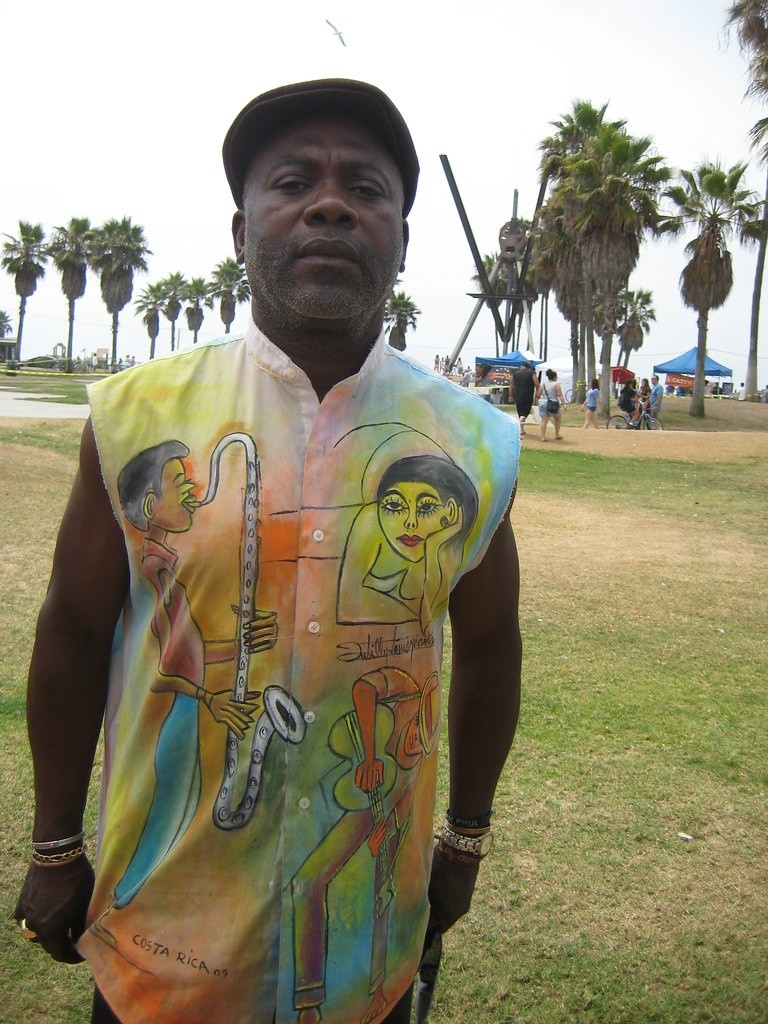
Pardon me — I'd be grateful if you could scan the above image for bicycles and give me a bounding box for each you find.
[606,397,663,431]
[565,389,573,403]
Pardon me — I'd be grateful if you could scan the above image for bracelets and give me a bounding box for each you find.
[434,808,493,864]
[31,829,87,867]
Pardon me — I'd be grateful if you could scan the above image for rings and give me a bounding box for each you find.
[19,918,37,938]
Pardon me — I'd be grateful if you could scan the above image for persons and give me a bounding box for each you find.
[77,353,135,375]
[508,362,568,442]
[13,79,522,1024]
[665,380,768,404]
[434,354,474,387]
[618,375,663,425]
[581,378,602,429]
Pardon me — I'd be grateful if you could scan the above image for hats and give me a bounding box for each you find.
[222,78,421,217]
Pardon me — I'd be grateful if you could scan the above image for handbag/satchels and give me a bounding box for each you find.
[547,399,560,413]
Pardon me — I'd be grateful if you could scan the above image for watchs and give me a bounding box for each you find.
[442,825,493,857]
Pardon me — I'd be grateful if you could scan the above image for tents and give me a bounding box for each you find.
[475,349,542,404]
[653,346,733,401]
[610,366,635,398]
[520,351,552,403]
